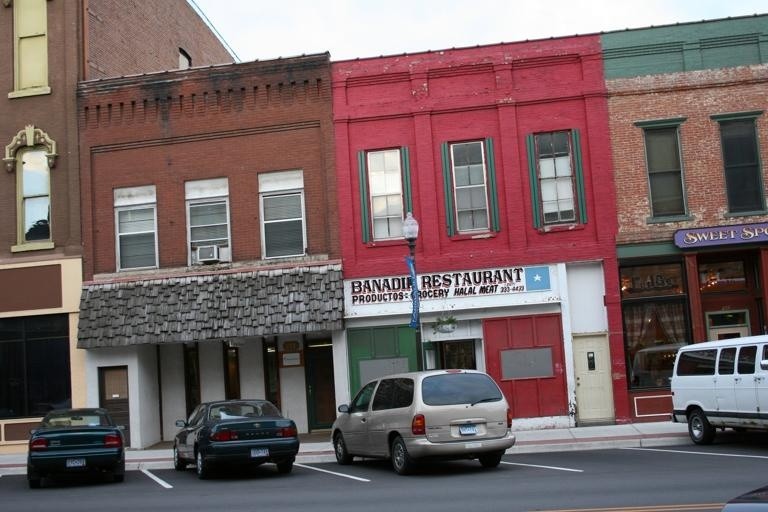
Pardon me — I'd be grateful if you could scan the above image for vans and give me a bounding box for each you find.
[330,369,516,475]
[670,335,768,445]
[632,342,688,388]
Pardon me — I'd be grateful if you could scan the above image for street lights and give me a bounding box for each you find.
[402,212,423,371]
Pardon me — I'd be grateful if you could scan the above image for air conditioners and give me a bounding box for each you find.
[196,244,221,263]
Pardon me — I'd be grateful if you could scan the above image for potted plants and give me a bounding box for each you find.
[431,316,459,334]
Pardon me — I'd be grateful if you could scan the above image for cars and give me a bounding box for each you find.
[173,399,299,479]
[27,408,125,489]
